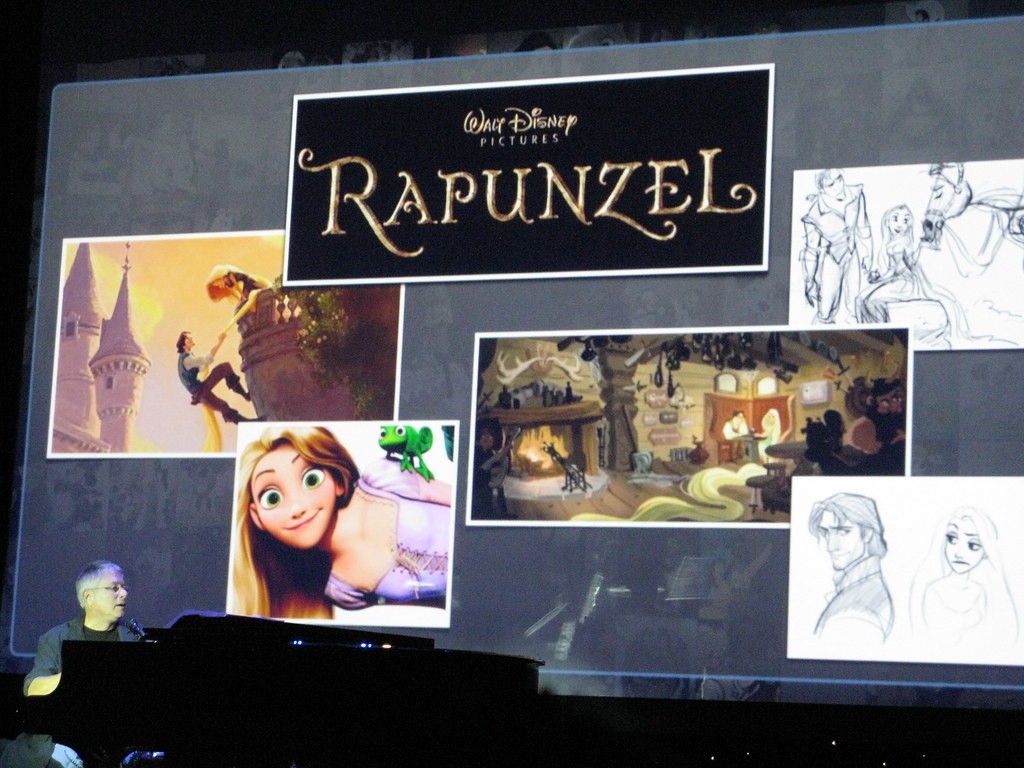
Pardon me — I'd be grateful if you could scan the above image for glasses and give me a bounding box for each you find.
[87,586,130,595]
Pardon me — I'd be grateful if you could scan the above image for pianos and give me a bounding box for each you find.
[18,614,548,768]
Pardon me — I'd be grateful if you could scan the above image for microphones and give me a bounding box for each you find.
[125,617,145,639]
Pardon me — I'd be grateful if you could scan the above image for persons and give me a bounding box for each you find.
[0,560,141,768]
